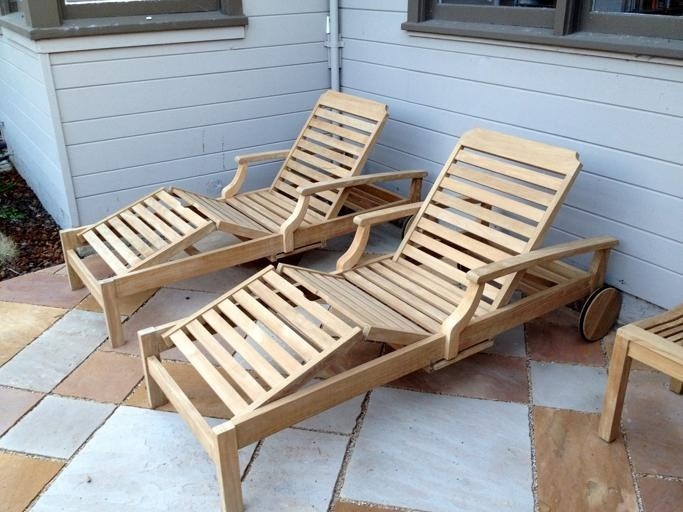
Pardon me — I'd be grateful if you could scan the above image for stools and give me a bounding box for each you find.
[597,300,683,443]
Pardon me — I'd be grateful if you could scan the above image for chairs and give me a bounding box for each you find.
[57,88,429,347]
[136,128,622,512]
